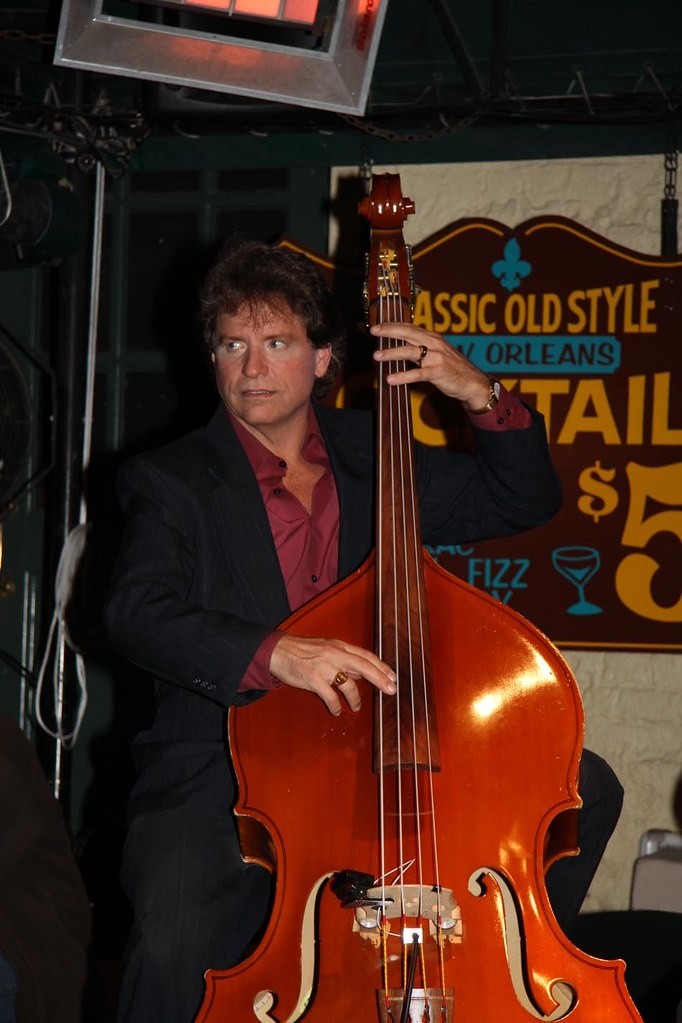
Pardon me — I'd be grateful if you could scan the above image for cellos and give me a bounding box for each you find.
[190,174,642,1023]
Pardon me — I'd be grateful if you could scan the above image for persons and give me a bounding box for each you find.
[104,242,626,1023]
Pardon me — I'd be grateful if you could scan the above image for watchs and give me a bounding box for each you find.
[467,380,501,415]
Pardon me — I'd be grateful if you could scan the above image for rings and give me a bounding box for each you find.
[334,671,348,687]
[417,345,428,363]
